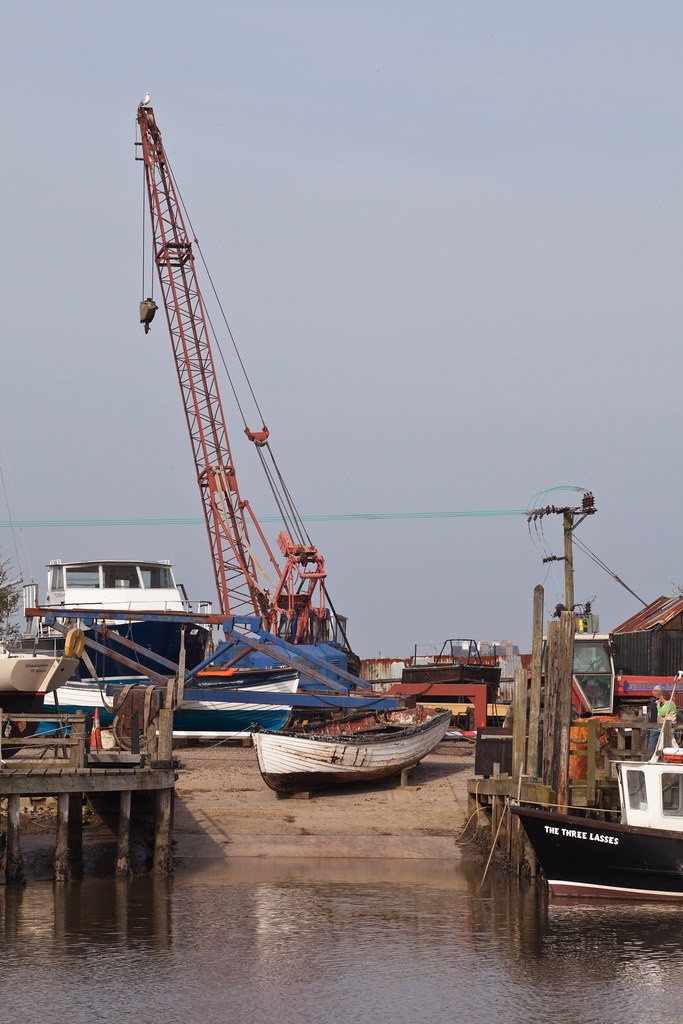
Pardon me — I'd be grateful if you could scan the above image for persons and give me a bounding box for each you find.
[644,685,676,761]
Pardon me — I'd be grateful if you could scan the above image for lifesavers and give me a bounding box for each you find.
[64,627,85,657]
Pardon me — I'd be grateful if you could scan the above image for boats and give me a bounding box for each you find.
[5,556,407,739]
[506,716,682,901]
[248,711,458,792]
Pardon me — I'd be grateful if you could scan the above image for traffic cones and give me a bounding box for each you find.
[82,706,107,755]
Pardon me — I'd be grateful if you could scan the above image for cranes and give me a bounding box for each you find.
[128,92,344,636]
